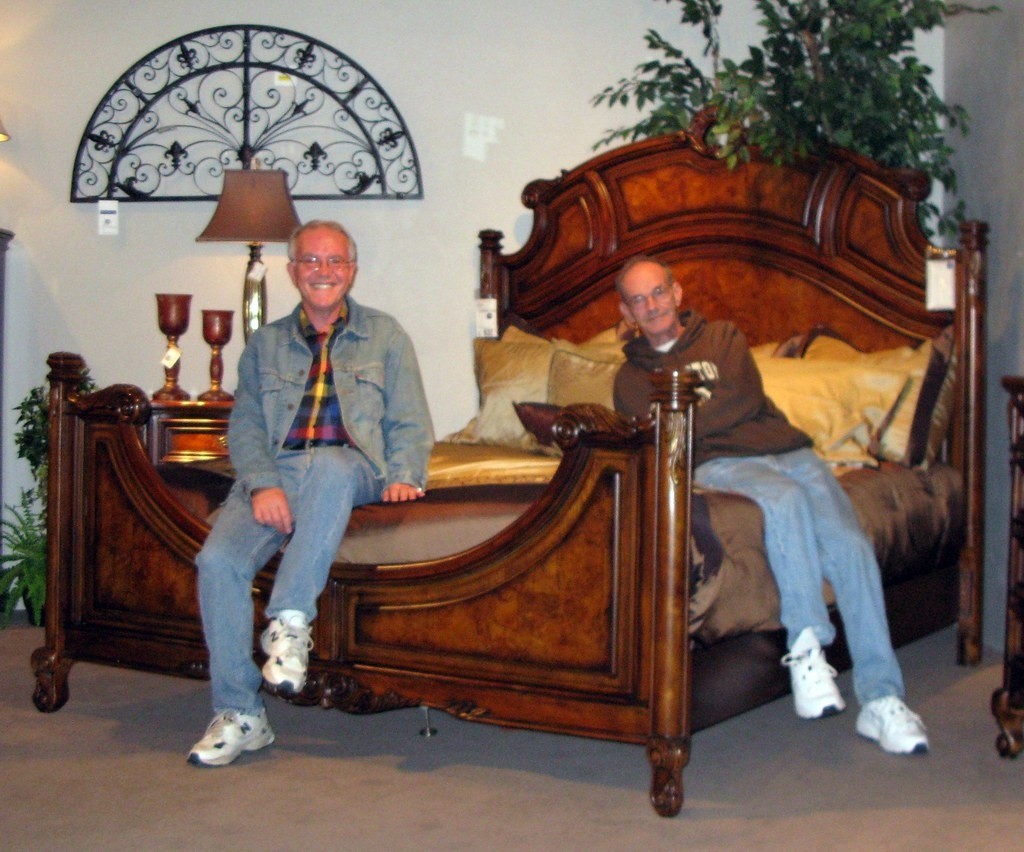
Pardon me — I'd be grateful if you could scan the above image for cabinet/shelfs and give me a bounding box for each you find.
[139,401,235,466]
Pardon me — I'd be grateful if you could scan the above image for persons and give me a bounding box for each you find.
[613,257,930,757]
[186,218,436,768]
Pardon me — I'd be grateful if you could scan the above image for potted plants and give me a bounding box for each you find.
[0,486,47,629]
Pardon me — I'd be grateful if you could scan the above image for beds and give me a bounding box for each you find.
[27,98,989,817]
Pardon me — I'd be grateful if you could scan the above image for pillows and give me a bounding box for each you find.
[436,321,960,472]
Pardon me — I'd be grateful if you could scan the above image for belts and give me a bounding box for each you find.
[291,438,342,451]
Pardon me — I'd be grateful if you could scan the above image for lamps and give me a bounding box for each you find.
[193,168,304,345]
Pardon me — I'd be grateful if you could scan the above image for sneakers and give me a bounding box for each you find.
[781,626,846,721]
[186,706,276,767]
[260,613,315,698]
[854,693,930,755]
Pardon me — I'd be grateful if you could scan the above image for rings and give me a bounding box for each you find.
[417,488,422,493]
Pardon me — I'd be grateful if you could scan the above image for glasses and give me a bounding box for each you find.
[291,254,350,272]
[627,284,675,310]
